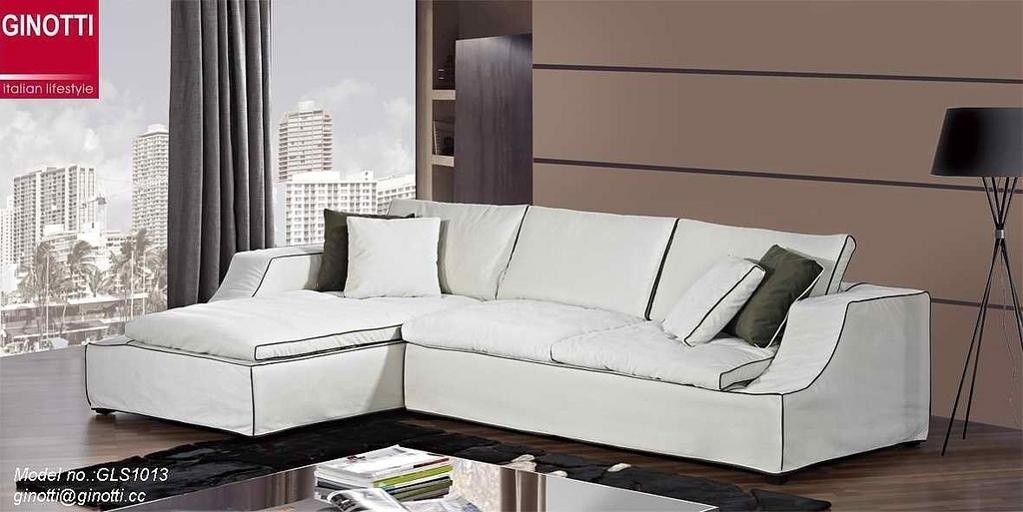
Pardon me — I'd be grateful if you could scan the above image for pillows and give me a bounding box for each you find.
[342,216,444,299]
[722,244,824,348]
[386,198,528,300]
[314,208,416,292]
[648,216,857,322]
[662,254,766,348]
[495,206,680,319]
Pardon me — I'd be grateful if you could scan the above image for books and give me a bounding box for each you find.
[313,443,452,501]
[307,485,479,512]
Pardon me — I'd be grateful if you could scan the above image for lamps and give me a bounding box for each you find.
[930,106,1023,458]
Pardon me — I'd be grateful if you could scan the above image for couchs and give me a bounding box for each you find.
[84,243,930,487]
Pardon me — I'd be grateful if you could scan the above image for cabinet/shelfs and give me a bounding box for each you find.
[418,0,456,201]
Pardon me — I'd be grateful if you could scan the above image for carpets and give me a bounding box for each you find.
[17,416,830,512]
